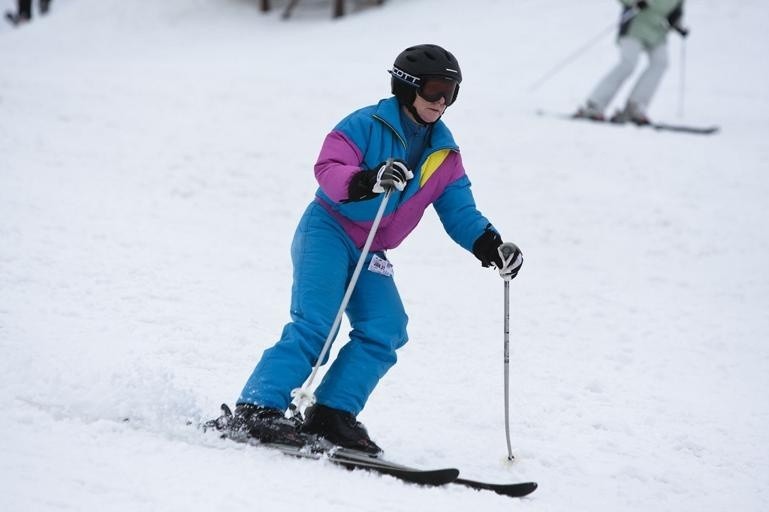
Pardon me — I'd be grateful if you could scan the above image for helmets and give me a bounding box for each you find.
[390,44,463,107]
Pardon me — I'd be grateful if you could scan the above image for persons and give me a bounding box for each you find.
[230,39,528,456]
[9,1,52,25]
[570,0,692,130]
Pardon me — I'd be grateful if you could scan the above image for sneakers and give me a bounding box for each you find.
[229,402,325,453]
[301,403,384,457]
[576,105,651,125]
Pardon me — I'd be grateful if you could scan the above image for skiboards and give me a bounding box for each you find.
[538,110,719,134]
[124,415,538,497]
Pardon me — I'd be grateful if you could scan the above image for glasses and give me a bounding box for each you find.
[416,73,457,106]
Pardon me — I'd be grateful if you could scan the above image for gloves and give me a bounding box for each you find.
[347,158,414,203]
[472,229,524,281]
